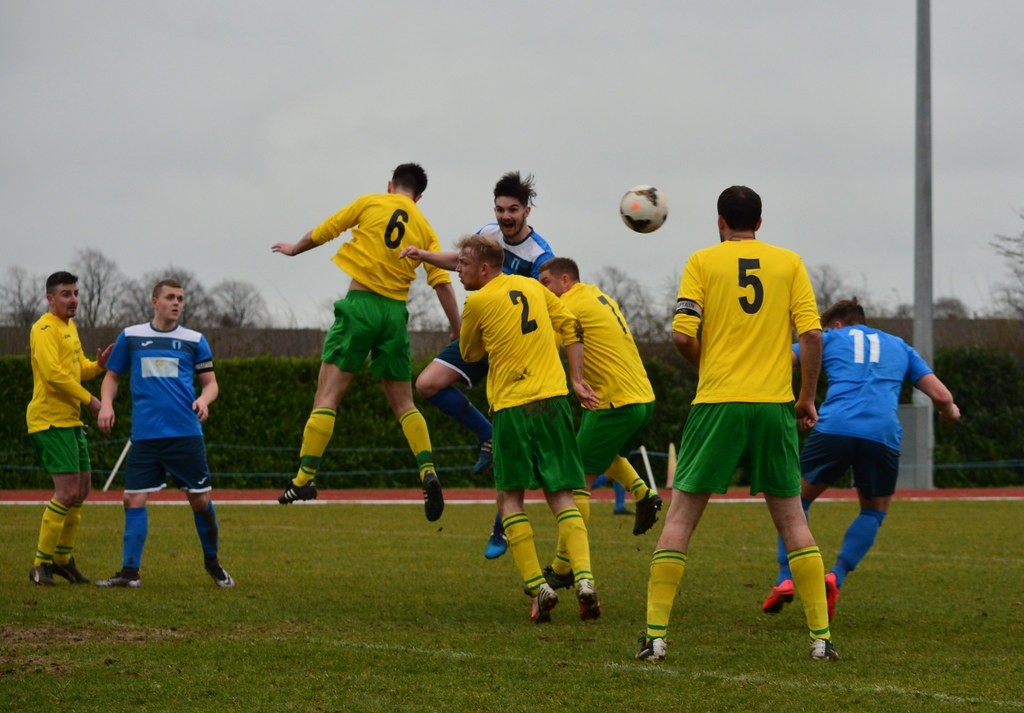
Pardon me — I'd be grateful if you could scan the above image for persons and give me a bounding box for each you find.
[634,187,844,662]
[763,295,959,622]
[95,279,240,588]
[456,236,602,626]
[271,162,461,521]
[534,256,663,590]
[398,172,555,559]
[27,272,115,587]
[594,475,637,516]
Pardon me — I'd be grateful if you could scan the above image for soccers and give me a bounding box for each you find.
[619,184,669,234]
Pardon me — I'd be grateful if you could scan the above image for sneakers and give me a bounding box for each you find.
[529,583,558,625]
[632,488,663,535]
[278,479,317,504]
[824,572,841,622]
[422,470,445,522]
[29,562,56,586]
[484,513,508,559]
[524,566,575,596]
[95,571,140,588]
[613,507,636,516]
[809,638,839,662]
[472,438,494,476]
[762,579,794,614]
[52,557,90,584]
[203,562,236,588]
[633,631,670,663]
[574,579,601,621]
